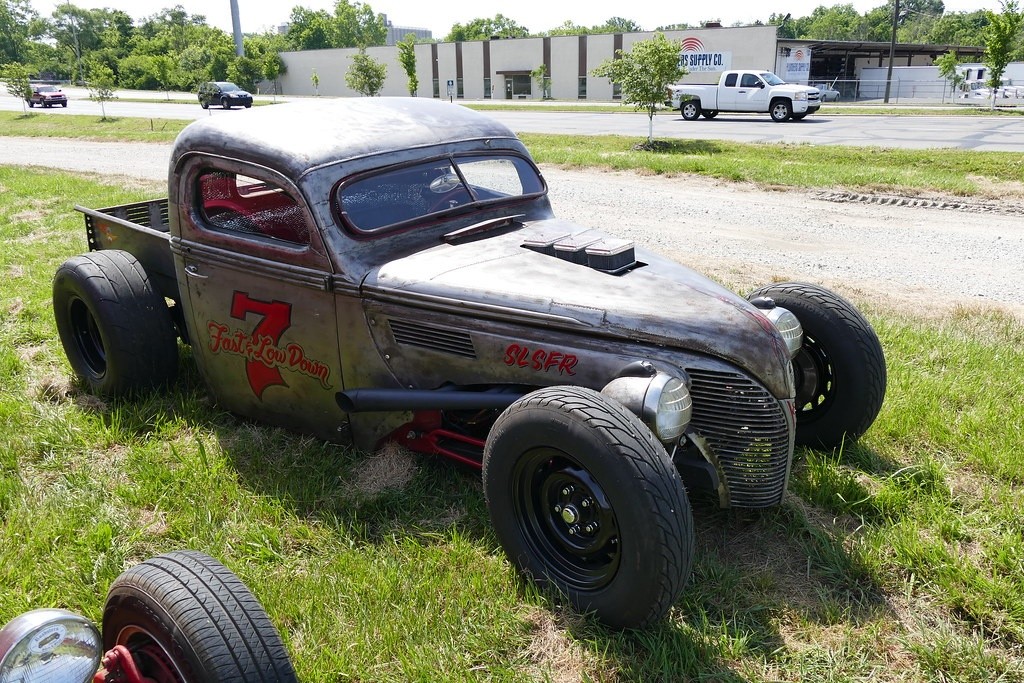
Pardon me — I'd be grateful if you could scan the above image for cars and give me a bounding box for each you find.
[809,83,841,103]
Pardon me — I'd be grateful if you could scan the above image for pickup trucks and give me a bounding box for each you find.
[54,95,888,636]
[664,70,822,122]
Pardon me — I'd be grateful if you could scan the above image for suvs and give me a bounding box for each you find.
[24,85,67,108]
[198,82,254,109]
